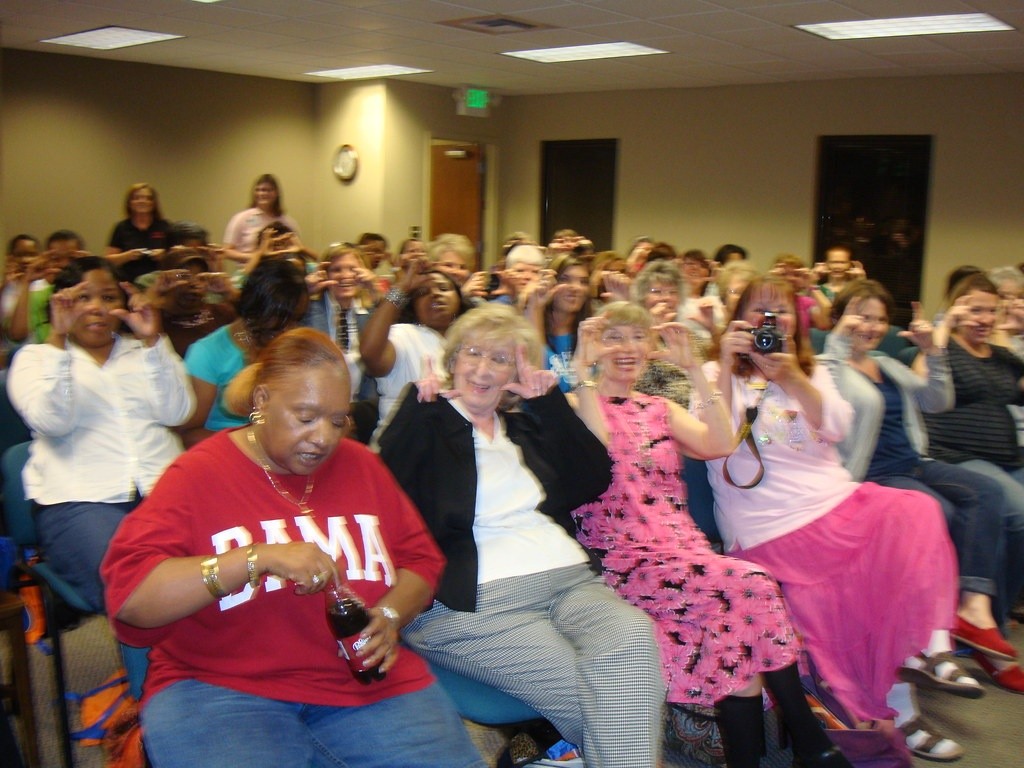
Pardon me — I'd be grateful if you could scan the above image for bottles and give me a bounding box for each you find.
[323,576,387,685]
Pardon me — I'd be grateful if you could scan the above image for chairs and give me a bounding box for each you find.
[680,453,724,544]
[0,369,149,767]
[399,642,546,728]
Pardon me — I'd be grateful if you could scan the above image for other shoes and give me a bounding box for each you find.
[949,616,1019,662]
[794,745,854,768]
[971,644,1024,696]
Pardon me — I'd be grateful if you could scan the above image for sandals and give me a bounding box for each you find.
[900,713,962,761]
[897,647,985,699]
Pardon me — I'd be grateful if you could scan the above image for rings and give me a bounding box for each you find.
[311,575,322,584]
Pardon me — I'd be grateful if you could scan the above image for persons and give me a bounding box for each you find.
[378,300,662,768]
[689,276,985,768]
[100,328,490,768]
[1,174,1024,768]
[567,306,853,768]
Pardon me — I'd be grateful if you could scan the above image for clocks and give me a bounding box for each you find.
[332,144,359,182]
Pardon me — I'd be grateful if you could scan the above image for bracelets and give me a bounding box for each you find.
[809,286,821,290]
[199,554,230,599]
[246,543,260,589]
[695,390,721,410]
[380,606,400,630]
[382,288,406,309]
[573,380,598,390]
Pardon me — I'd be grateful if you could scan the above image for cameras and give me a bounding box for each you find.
[738,311,783,360]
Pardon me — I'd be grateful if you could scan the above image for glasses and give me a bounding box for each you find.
[457,348,517,370]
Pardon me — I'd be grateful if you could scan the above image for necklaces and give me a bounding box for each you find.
[602,400,653,470]
[246,428,315,520]
